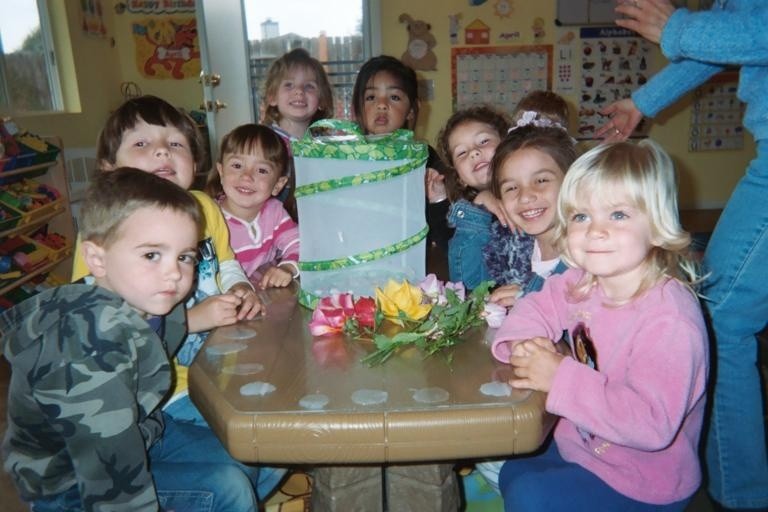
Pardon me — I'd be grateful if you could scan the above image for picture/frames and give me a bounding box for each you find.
[450,43,554,116]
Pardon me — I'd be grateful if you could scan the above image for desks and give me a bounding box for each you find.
[188,257,574,512]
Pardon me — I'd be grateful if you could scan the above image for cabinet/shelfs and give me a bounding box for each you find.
[0,137,74,296]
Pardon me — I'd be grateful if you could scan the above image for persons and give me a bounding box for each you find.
[448,111,580,498]
[508,88,571,132]
[196,121,301,293]
[70,93,291,504]
[255,47,336,155]
[1,167,265,510]
[489,139,710,509]
[434,105,518,294]
[351,56,457,252]
[591,1,768,511]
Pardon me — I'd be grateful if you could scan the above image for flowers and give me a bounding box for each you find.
[311,275,497,376]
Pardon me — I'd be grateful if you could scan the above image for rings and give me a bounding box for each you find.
[634,0,644,8]
[614,129,620,132]
